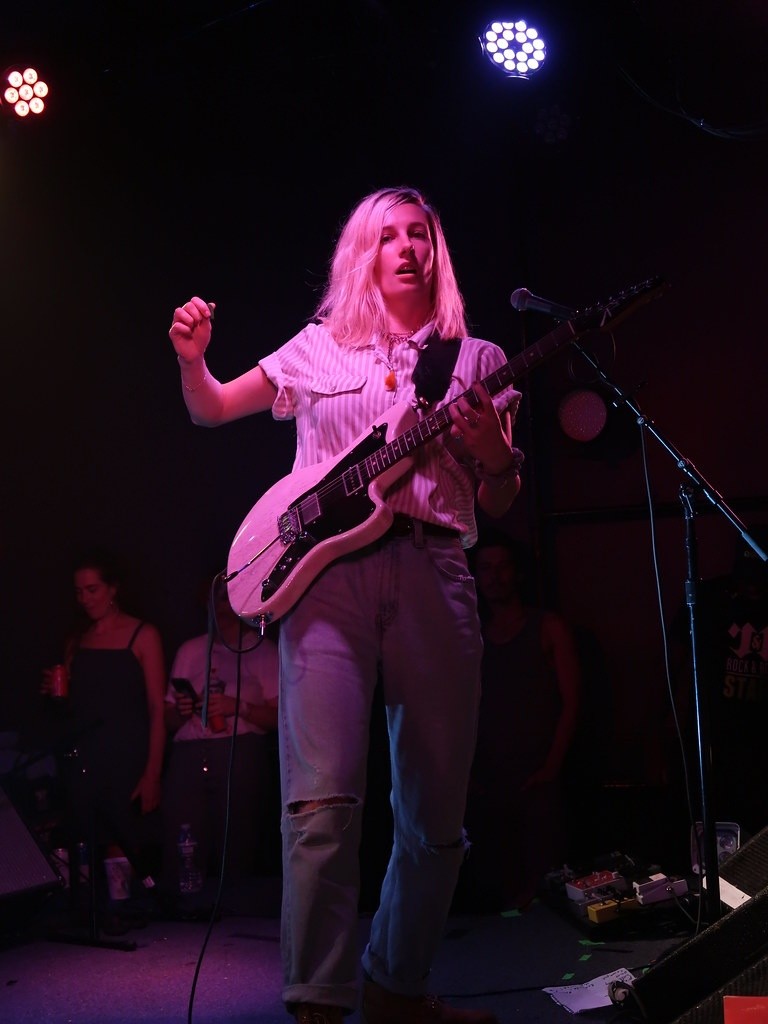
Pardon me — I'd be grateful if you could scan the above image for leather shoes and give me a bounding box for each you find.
[361,982,498,1024]
[296,1003,343,1024]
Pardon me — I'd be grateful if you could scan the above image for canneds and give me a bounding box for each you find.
[50,664,69,698]
[54,849,70,887]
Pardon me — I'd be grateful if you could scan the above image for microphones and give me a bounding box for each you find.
[511,288,580,320]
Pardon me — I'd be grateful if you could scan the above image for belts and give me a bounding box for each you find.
[388,512,459,537]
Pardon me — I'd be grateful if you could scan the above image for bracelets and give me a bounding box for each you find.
[473,447,525,484]
[182,367,207,392]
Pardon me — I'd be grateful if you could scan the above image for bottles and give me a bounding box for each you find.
[51,664,69,699]
[177,823,205,892]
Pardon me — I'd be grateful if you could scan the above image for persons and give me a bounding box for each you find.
[168,571,281,910]
[661,524,766,824]
[468,536,585,906]
[40,557,166,907]
[162,181,529,1020]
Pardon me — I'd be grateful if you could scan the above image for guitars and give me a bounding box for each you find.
[229,273,675,628]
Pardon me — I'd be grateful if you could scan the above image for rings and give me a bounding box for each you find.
[472,414,480,424]
[456,434,463,440]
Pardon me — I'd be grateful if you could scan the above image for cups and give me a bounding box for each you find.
[104,856,131,899]
[208,668,227,733]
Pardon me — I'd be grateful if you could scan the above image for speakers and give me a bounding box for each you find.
[0,788,61,942]
[616,825,768,1024]
